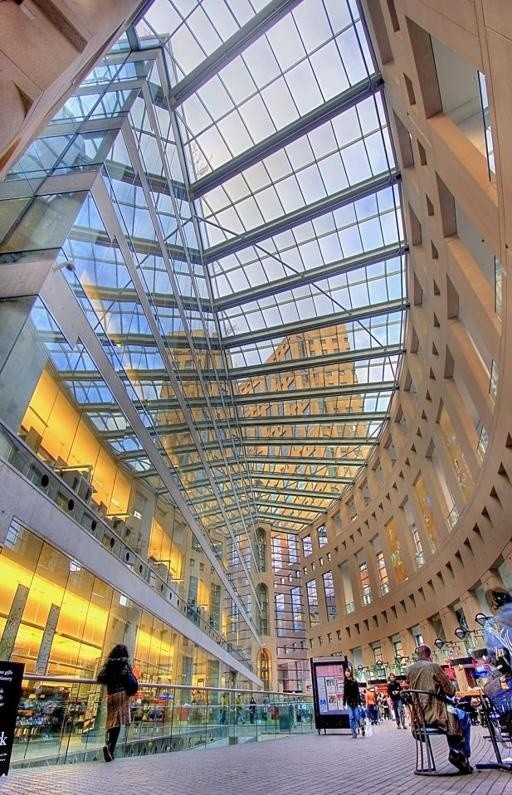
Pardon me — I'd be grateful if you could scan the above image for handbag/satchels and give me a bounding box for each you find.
[126,668,138,696]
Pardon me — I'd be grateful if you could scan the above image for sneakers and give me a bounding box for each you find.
[448,749,474,773]
[397,725,407,729]
[102,745,113,762]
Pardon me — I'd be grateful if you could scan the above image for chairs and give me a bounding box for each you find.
[399,688,467,777]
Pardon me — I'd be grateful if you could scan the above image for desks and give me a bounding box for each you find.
[460,689,512,771]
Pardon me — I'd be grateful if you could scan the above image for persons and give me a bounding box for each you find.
[249,697,257,724]
[235,695,246,724]
[341,591,511,776]
[220,696,228,726]
[97,643,139,763]
